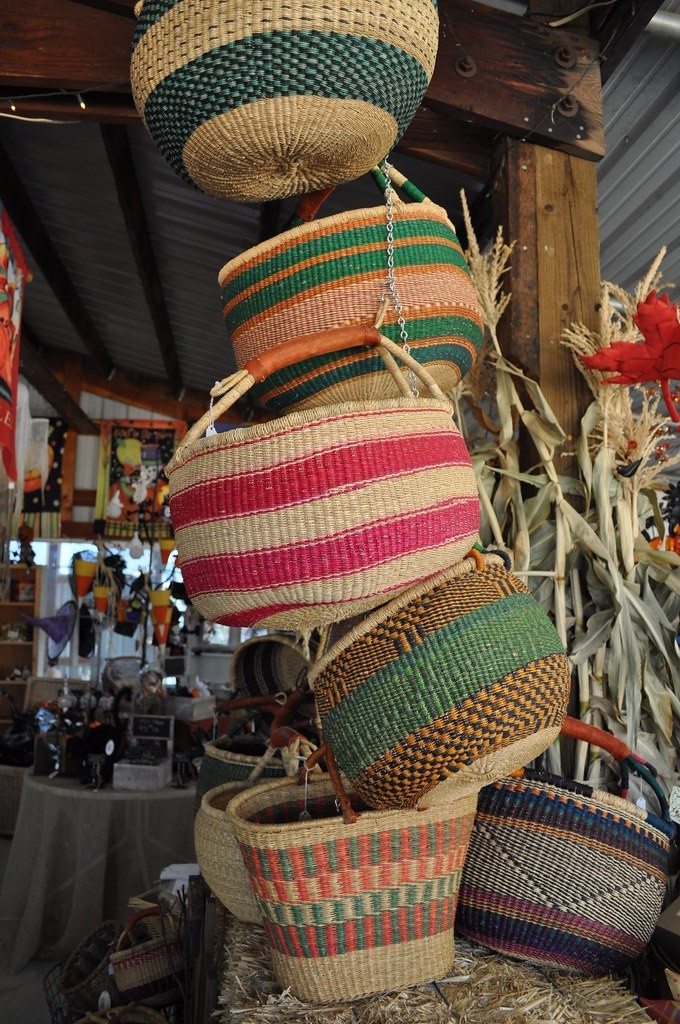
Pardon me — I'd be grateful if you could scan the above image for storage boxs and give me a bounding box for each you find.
[112,0,670,997]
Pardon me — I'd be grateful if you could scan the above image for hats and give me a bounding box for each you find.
[19,602,79,659]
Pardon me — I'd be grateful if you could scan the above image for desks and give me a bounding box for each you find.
[1,764,203,980]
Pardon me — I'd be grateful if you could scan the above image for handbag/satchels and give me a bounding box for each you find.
[43,0,675,1024]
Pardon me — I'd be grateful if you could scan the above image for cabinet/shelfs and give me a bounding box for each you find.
[0,561,56,760]
